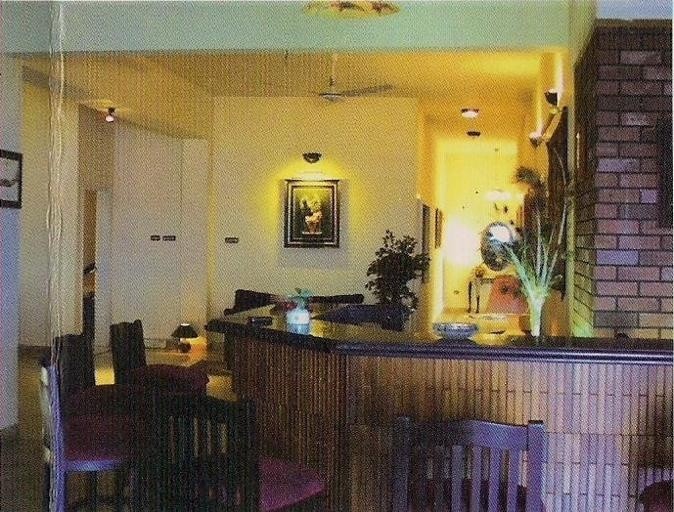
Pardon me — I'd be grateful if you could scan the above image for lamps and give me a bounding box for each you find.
[170,321,199,353]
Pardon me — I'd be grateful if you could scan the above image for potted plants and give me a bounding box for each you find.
[492,138,578,344]
[366,227,431,333]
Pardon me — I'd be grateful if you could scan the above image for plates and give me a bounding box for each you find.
[431,321,480,338]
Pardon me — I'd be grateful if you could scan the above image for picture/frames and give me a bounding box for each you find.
[0,146,24,208]
[284,177,343,251]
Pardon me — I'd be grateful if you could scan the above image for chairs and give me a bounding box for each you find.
[389,414,547,512]
[49,334,136,512]
[109,318,208,392]
[128,377,326,510]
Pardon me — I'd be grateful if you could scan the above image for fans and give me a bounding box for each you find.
[277,52,396,108]
